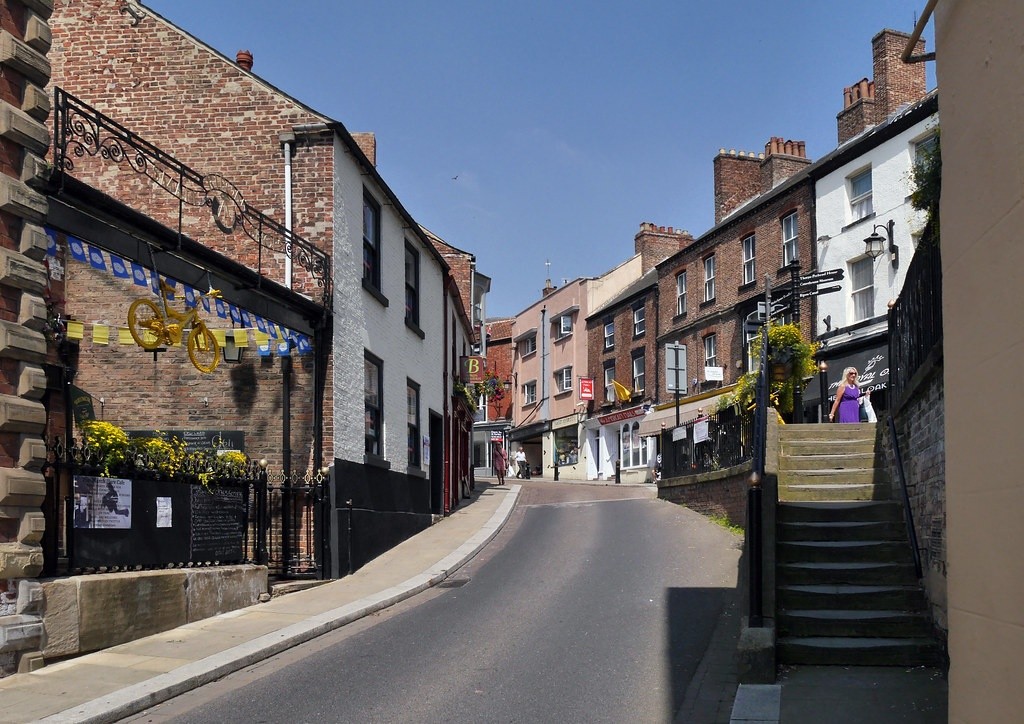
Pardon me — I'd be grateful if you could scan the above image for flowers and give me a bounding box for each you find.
[477,368,504,405]
[748,321,809,363]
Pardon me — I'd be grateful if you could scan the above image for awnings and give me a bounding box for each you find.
[638,392,733,437]
[800,339,890,406]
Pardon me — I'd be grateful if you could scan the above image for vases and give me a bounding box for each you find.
[773,361,789,382]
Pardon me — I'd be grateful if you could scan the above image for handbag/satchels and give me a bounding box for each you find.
[859,396,878,424]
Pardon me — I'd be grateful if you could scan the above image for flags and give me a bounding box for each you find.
[612,380,632,402]
[46,229,313,357]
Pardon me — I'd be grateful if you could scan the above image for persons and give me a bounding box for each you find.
[493,442,507,485]
[829,365,870,423]
[515,446,527,478]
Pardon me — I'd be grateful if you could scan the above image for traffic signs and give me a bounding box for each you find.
[800,268,844,298]
[770,290,794,320]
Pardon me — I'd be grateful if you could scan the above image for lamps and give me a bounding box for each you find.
[219,310,250,366]
[503,371,518,393]
[863,220,897,267]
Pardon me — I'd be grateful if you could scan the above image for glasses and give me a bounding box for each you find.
[496,444,500,445]
[850,373,856,376]
